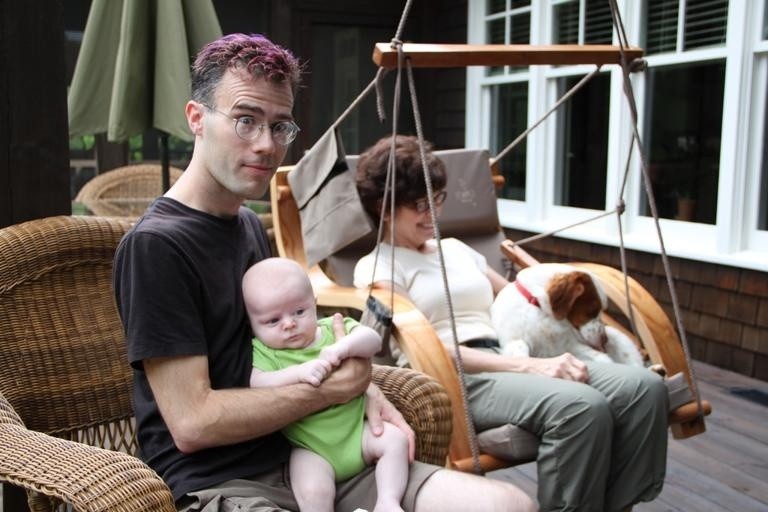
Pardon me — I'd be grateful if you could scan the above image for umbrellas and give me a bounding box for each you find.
[67,0,225,193]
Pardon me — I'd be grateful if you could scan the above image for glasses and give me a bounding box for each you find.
[405,191,447,212]
[203,104,302,146]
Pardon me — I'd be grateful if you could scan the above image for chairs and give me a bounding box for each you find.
[0,215,452,512]
[73,163,275,235]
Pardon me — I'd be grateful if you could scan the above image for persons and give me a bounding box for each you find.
[242,257,409,512]
[352,135,669,512]
[112,33,538,512]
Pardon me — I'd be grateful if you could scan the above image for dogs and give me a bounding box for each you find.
[490,263,667,376]
